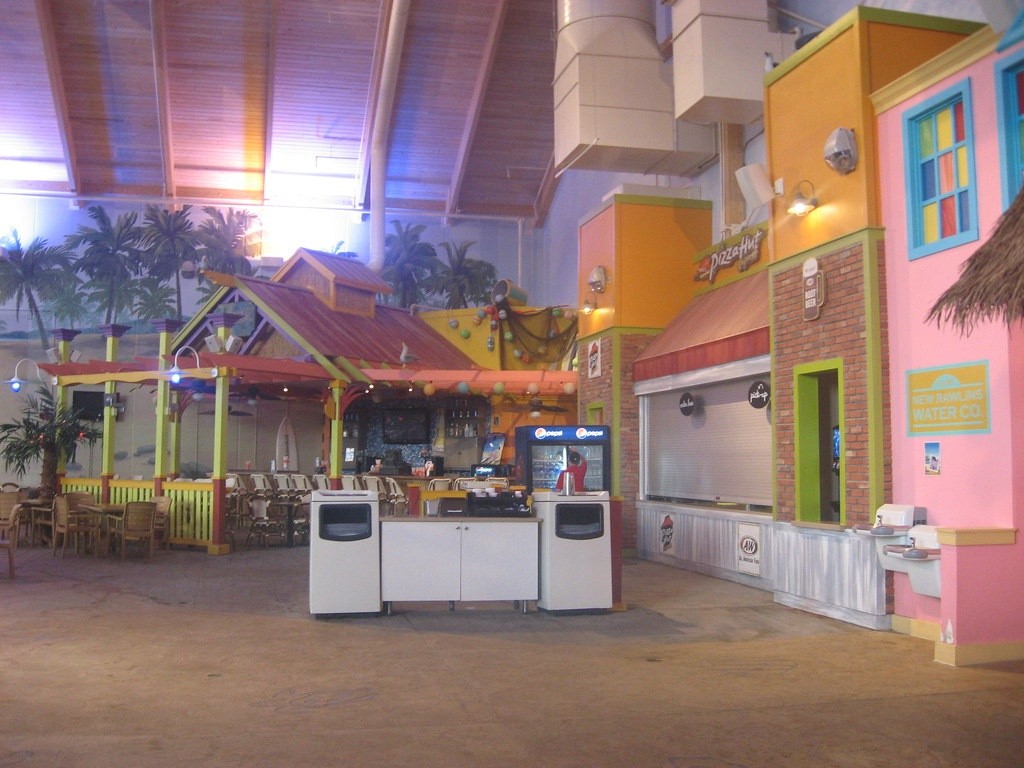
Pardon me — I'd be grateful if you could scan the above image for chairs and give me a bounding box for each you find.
[226,474,511,550]
[0,482,172,577]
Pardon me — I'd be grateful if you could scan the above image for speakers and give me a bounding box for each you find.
[735,163,776,210]
[204,334,223,352]
[225,334,242,355]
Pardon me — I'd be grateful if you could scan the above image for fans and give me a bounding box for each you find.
[229,387,280,401]
[170,381,215,394]
[506,395,569,418]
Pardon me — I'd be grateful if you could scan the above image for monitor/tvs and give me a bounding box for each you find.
[382,407,431,444]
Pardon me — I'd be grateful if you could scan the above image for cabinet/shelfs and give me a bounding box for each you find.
[340,406,366,468]
[443,396,486,470]
[382,518,542,615]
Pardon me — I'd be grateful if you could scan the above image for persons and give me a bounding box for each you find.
[555,446,587,492]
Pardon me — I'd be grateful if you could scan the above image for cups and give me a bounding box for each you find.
[563,472,575,496]
[472,488,482,496]
[489,493,498,497]
[485,487,495,493]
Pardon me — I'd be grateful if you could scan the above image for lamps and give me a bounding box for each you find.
[588,264,606,293]
[164,345,218,384]
[2,358,59,392]
[191,380,205,402]
[787,180,817,216]
[530,410,541,418]
[822,126,858,175]
[247,394,259,405]
[584,300,593,314]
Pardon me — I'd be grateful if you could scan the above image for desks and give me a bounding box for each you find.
[22,498,54,549]
[271,501,309,548]
[421,491,468,519]
[80,503,124,557]
